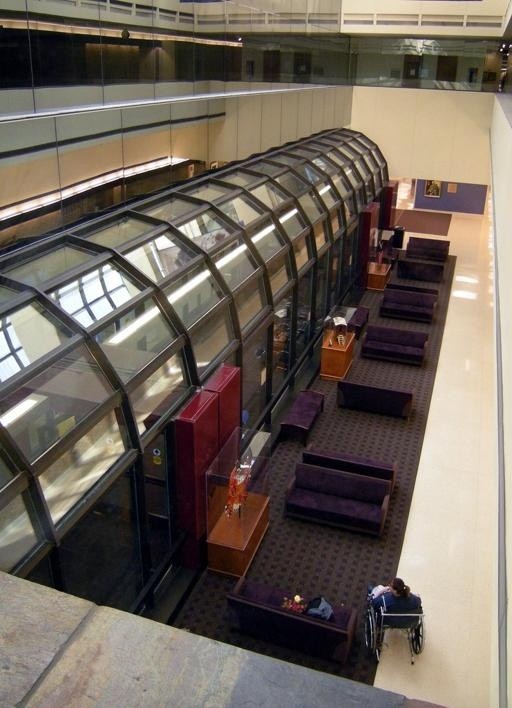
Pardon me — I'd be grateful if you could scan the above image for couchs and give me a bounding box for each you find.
[223,575,356,664]
[343,225,454,369]
[281,462,392,537]
[302,442,399,495]
[334,379,414,421]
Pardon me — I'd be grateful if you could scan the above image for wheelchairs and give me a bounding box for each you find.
[363,581,427,666]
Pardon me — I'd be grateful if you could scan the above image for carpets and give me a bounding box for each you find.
[167,249,457,690]
[396,208,453,237]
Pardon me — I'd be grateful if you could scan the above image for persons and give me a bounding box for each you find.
[368,578,422,620]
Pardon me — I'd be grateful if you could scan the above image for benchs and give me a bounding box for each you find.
[279,388,326,448]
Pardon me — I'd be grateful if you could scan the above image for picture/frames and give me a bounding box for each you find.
[423,180,443,198]
